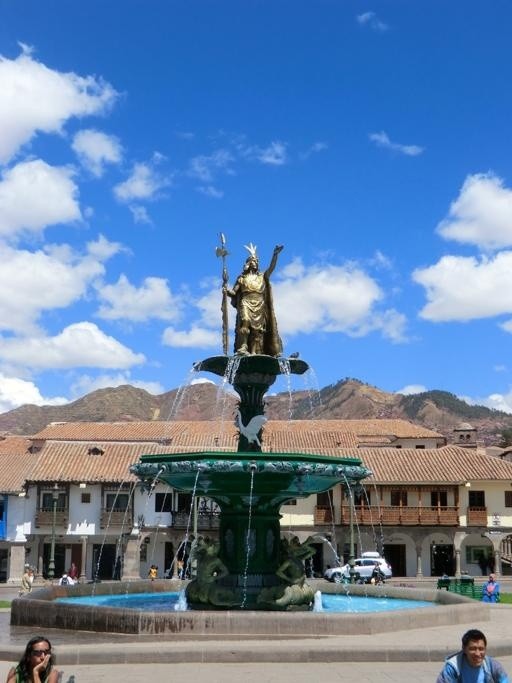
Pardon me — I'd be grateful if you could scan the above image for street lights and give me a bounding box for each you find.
[45,482,61,580]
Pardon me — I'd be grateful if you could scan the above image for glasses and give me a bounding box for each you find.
[31,649,52,657]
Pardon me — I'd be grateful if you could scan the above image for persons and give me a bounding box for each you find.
[147,564,161,579]
[68,562,79,578]
[23,566,35,593]
[441,570,449,590]
[59,570,74,585]
[461,570,472,578]
[369,561,385,583]
[222,242,283,356]
[435,628,509,683]
[7,636,59,683]
[457,570,465,578]
[480,572,499,603]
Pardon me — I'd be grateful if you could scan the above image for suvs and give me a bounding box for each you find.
[323,549,395,585]
